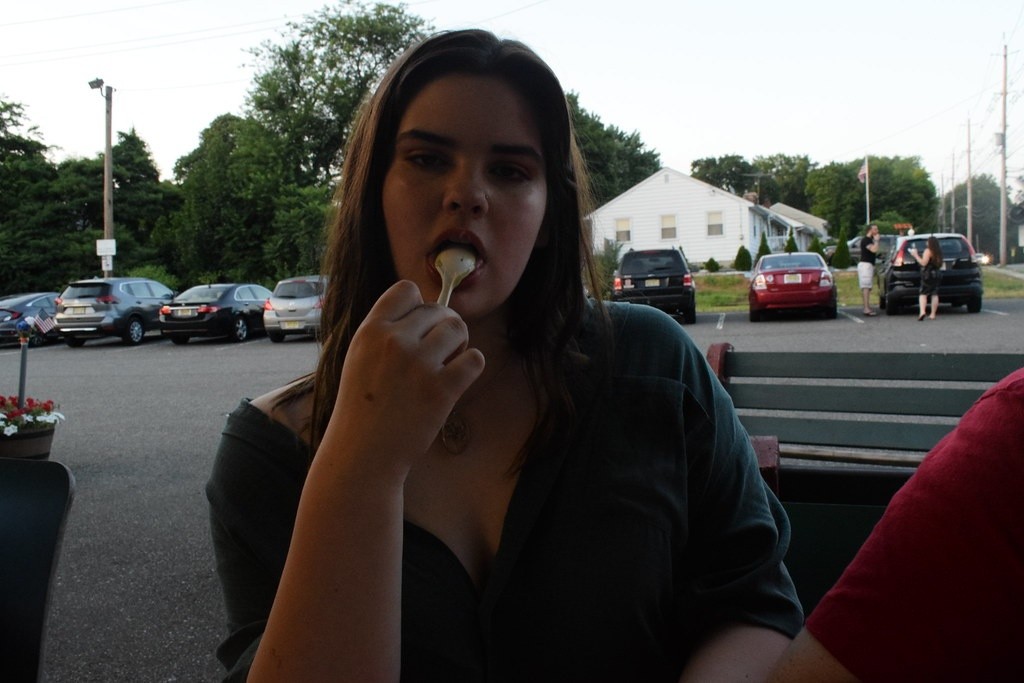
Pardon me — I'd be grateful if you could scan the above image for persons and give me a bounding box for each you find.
[765,368,1024,683]
[205,30,804,683]
[858,224,881,316]
[910,237,942,321]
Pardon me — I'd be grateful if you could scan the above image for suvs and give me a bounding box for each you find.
[55,275,174,348]
[263,274,328,343]
[610,247,697,324]
[877,236,984,313]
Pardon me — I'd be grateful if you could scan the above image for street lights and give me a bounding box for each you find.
[89,77,115,277]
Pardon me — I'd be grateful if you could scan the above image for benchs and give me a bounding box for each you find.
[708,338,1024,613]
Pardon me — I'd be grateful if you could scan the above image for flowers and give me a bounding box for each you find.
[0,394,66,437]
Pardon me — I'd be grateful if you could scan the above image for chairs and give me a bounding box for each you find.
[0,458,76,683]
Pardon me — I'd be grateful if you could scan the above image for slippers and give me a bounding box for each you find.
[862,309,877,316]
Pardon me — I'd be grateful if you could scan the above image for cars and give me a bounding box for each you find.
[160,282,273,343]
[821,237,862,262]
[0,292,62,349]
[745,251,836,322]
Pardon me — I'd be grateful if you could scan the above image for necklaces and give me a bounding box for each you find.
[440,358,512,454]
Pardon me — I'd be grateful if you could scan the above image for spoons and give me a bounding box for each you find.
[435,245,476,307]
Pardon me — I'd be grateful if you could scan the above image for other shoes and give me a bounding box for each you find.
[918,313,936,322]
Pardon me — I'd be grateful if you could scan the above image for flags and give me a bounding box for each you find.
[858,161,866,184]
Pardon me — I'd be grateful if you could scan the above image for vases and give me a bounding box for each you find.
[0,421,56,461]
